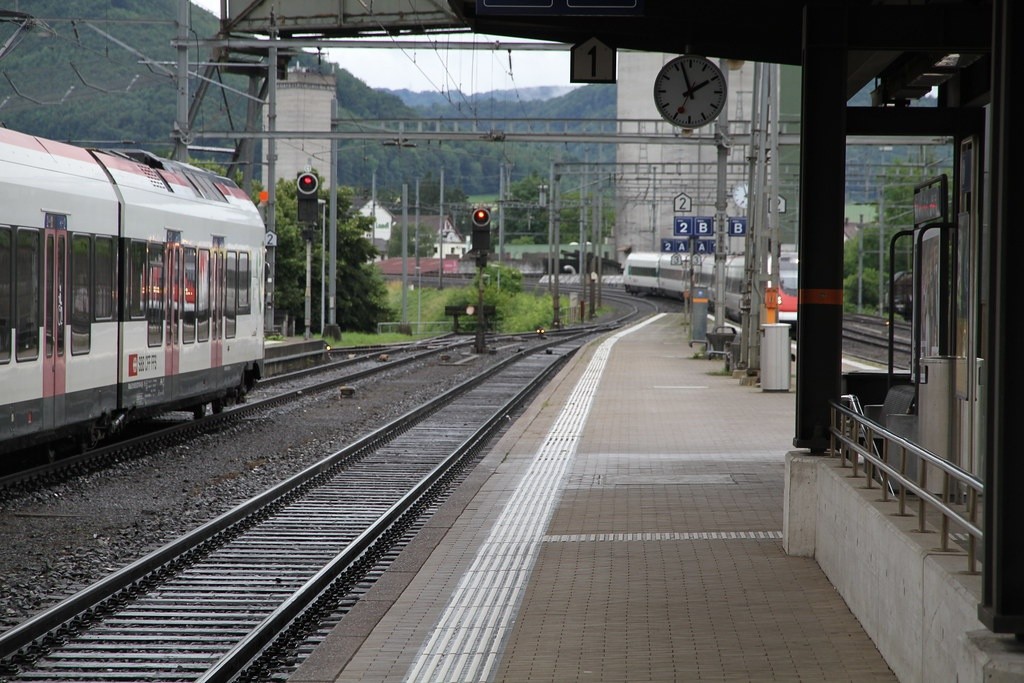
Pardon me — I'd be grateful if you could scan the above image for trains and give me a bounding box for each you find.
[0,126,266,450]
[623,243,798,324]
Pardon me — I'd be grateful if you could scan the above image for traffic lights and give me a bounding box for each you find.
[297,172,318,222]
[472,207,490,250]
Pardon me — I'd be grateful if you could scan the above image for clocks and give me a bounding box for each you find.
[653,45,727,128]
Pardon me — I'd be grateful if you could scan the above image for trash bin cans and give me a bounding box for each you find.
[759,323,790,392]
